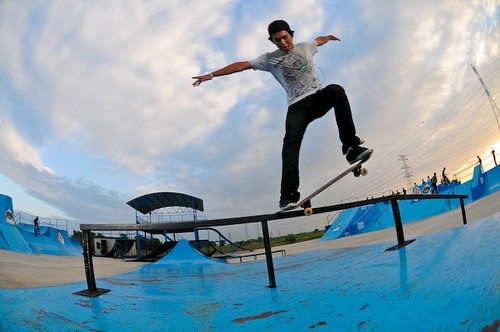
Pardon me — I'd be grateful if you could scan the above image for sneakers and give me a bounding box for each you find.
[279,199,297,209]
[346,146,371,165]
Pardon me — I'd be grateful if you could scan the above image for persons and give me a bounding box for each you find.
[402,188,407,195]
[491,148,498,166]
[392,191,395,195]
[113,243,123,259]
[395,190,399,195]
[413,182,417,187]
[191,20,373,213]
[430,173,438,194]
[442,167,447,186]
[477,155,484,173]
[421,179,425,182]
[428,176,429,179]
[34,216,40,234]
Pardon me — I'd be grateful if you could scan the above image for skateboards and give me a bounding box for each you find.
[279,149,373,215]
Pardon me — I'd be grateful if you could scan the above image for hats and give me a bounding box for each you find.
[268,20,289,34]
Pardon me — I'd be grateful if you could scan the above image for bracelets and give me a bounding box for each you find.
[209,73,214,80]
